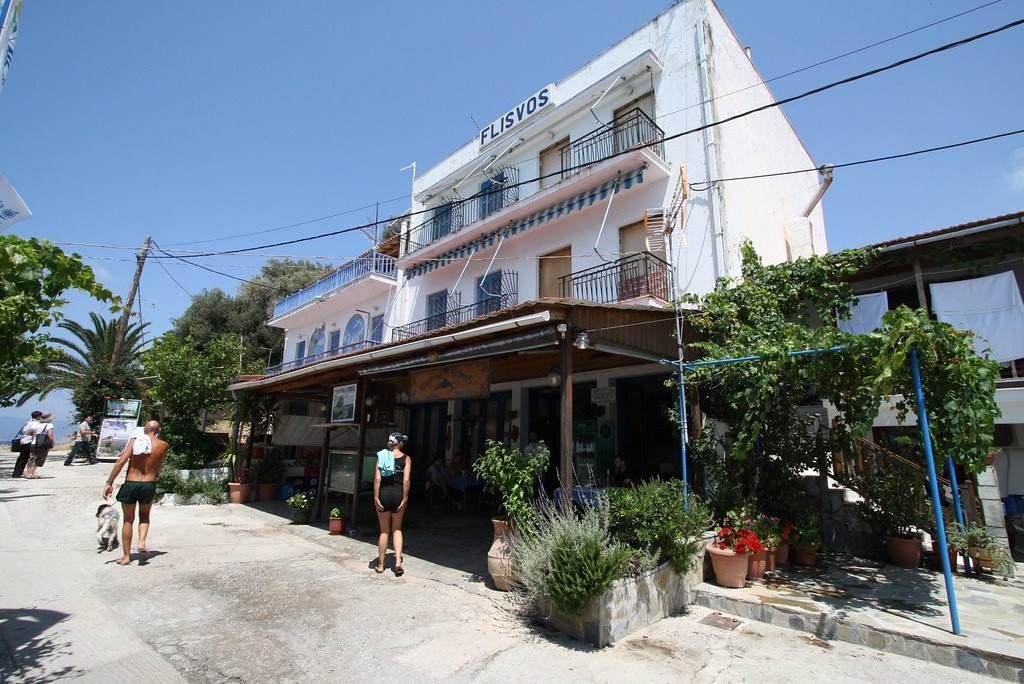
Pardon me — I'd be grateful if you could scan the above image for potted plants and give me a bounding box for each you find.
[964,522,1018,577]
[258,470,278,502]
[930,516,969,573]
[860,468,926,567]
[980,446,1003,465]
[476,437,717,648]
[329,505,346,534]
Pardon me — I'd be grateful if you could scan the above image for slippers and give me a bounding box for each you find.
[116,558,130,565]
[137,547,150,556]
[375,565,383,573]
[395,568,404,574]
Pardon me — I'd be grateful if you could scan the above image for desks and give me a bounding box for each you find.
[449,475,484,514]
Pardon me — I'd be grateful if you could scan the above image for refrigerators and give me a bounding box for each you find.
[571,419,616,487]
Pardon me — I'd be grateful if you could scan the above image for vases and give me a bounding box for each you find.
[776,544,788,566]
[747,547,768,578]
[704,543,749,588]
[289,507,311,522]
[765,546,777,571]
[226,482,254,504]
[793,545,818,566]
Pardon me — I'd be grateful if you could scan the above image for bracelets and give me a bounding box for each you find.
[106,480,113,485]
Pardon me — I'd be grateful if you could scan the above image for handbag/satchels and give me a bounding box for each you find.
[11,438,23,452]
[34,434,53,450]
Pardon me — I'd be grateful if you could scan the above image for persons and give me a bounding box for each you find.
[524,430,540,454]
[103,420,170,564]
[64,413,98,467]
[12,411,43,477]
[374,432,411,572]
[424,456,446,500]
[610,454,637,487]
[446,451,467,512]
[24,412,55,479]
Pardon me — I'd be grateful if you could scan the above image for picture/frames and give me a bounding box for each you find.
[330,379,359,423]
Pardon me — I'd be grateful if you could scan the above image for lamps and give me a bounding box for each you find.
[575,329,593,351]
[427,351,440,361]
[320,398,327,412]
[547,368,562,387]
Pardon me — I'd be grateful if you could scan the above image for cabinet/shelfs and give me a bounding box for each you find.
[311,423,401,531]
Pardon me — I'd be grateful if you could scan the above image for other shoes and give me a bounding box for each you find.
[25,474,39,479]
[13,474,24,478]
[67,464,74,466]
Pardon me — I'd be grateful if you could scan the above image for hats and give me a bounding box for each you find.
[389,432,408,445]
[40,412,55,423]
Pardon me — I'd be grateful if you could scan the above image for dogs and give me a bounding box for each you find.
[96,503,120,551]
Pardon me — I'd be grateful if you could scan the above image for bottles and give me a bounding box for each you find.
[366,407,371,423]
[575,436,594,458]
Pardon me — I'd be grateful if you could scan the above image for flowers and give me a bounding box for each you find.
[713,508,823,553]
[237,466,258,484]
[285,493,312,510]
